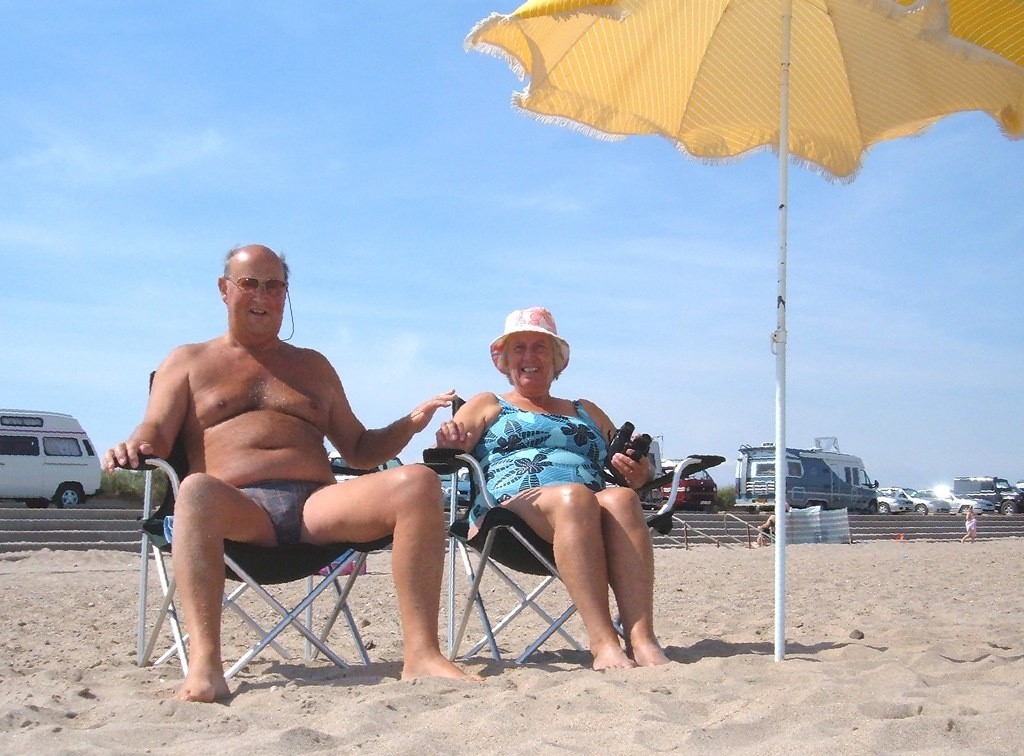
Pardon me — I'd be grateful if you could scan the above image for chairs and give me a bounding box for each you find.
[111,370,461,679]
[424,396,725,664]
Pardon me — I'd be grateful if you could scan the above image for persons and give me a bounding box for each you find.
[756,502,790,546]
[103,244,485,703]
[962,505,977,543]
[436,307,671,670]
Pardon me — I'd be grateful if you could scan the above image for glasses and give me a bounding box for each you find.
[228,277,288,297]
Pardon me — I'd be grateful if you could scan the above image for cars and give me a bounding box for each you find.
[326,450,403,485]
[439,473,472,511]
[876,490,913,515]
[910,487,995,516]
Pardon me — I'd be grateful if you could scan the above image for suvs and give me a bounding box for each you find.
[875,486,942,516]
[954,477,1024,516]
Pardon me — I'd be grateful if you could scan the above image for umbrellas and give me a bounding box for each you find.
[462,1,1024,662]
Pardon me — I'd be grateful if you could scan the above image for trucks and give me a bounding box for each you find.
[733,437,879,515]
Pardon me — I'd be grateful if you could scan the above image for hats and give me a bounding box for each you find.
[490,307,569,375]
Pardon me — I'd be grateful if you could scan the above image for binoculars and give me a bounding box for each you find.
[602,422,654,483]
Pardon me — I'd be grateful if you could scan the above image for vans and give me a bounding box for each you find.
[661,459,718,512]
[603,437,665,510]
[0,409,102,508]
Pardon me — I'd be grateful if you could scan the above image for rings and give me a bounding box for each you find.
[629,469,633,474]
